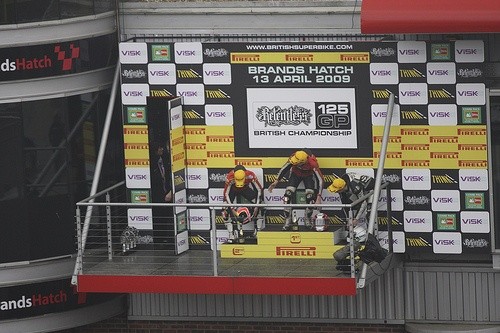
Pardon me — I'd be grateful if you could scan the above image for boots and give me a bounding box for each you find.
[303,207,313,230]
[253,219,264,239]
[224,222,238,244]
[282,209,292,231]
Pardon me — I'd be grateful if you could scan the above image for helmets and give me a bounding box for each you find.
[234,201,254,224]
[312,213,330,231]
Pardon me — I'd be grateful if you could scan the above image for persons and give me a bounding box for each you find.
[268,150,325,231]
[222,166,264,243]
[327,178,368,226]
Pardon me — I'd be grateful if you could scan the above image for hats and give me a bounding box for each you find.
[234,169,245,187]
[289,151,308,165]
[326,178,346,193]
